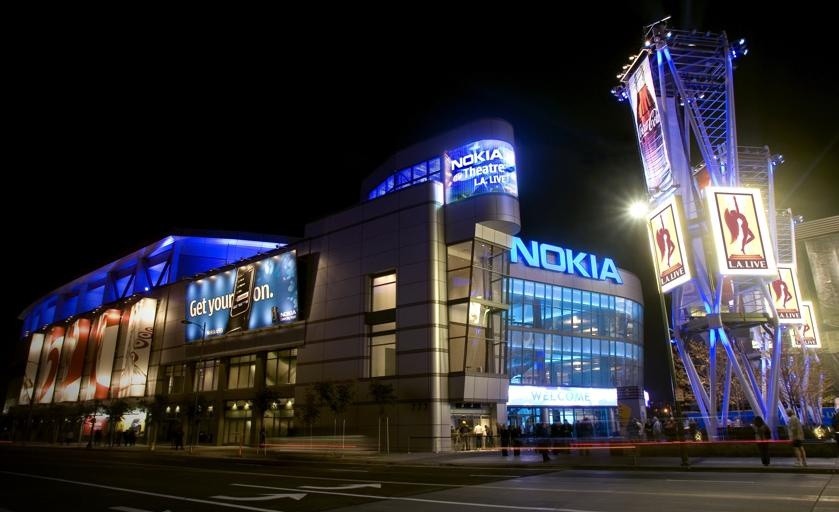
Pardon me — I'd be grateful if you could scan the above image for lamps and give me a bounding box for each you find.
[609,15,673,104]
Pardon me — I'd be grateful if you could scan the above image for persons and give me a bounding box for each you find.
[752,415,772,466]
[784,407,807,467]
[451,417,699,465]
[89,429,141,448]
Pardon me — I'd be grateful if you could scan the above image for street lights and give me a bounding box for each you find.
[179,316,209,455]
